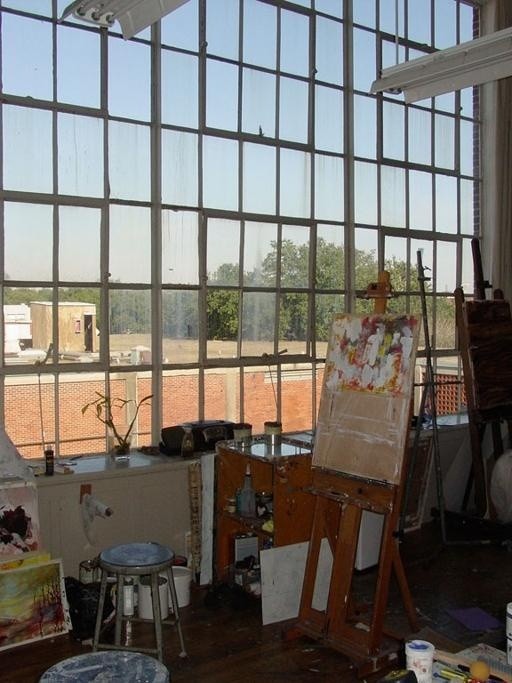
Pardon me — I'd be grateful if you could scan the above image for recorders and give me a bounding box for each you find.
[161,420,235,456]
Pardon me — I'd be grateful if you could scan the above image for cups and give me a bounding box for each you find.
[233,422,251,439]
[263,421,282,434]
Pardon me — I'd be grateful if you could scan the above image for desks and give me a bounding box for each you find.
[430,643,510,682]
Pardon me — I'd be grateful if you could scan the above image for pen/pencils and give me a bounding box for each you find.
[440,664,508,683]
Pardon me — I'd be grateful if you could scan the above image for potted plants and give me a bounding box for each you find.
[80,390,154,461]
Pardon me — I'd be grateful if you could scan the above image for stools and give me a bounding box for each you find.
[84,542,188,663]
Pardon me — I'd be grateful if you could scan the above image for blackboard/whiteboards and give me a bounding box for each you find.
[463,300,512,423]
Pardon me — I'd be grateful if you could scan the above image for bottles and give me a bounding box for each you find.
[238,461,256,518]
[181,423,195,458]
[120,575,134,617]
[43,450,54,476]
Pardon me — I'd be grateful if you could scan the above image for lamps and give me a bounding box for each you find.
[368,1,511,104]
[59,0,191,39]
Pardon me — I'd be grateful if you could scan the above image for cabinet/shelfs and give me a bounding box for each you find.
[406,411,470,516]
[212,441,340,603]
[0,447,190,590]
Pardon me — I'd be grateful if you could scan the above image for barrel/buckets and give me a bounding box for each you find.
[136,576,171,620]
[154,566,190,609]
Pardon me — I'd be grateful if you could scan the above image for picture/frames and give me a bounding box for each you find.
[401,432,435,534]
